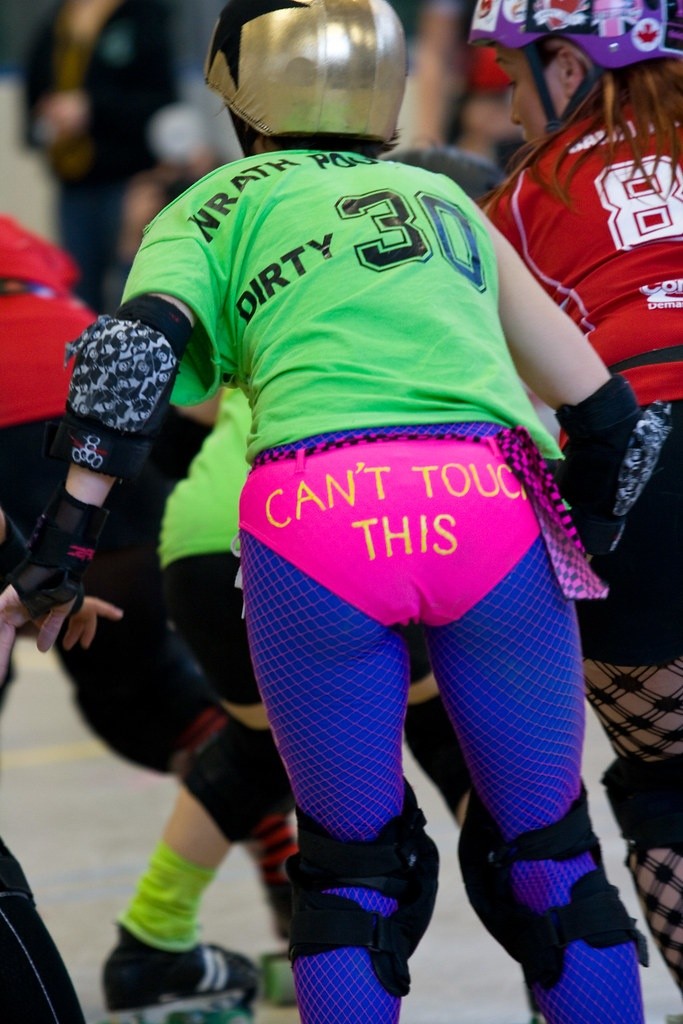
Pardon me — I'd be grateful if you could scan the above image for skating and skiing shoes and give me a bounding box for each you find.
[100,921,258,1024]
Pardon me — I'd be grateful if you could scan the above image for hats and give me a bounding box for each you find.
[150,109,213,172]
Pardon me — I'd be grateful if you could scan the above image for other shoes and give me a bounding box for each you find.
[262,944,303,1005]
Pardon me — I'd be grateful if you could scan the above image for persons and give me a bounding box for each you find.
[2,2,538,1023]
[0,0,656,1024]
[473,0,682,993]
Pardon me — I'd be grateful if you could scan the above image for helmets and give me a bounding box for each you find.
[205,0,407,144]
[469,0,667,69]
